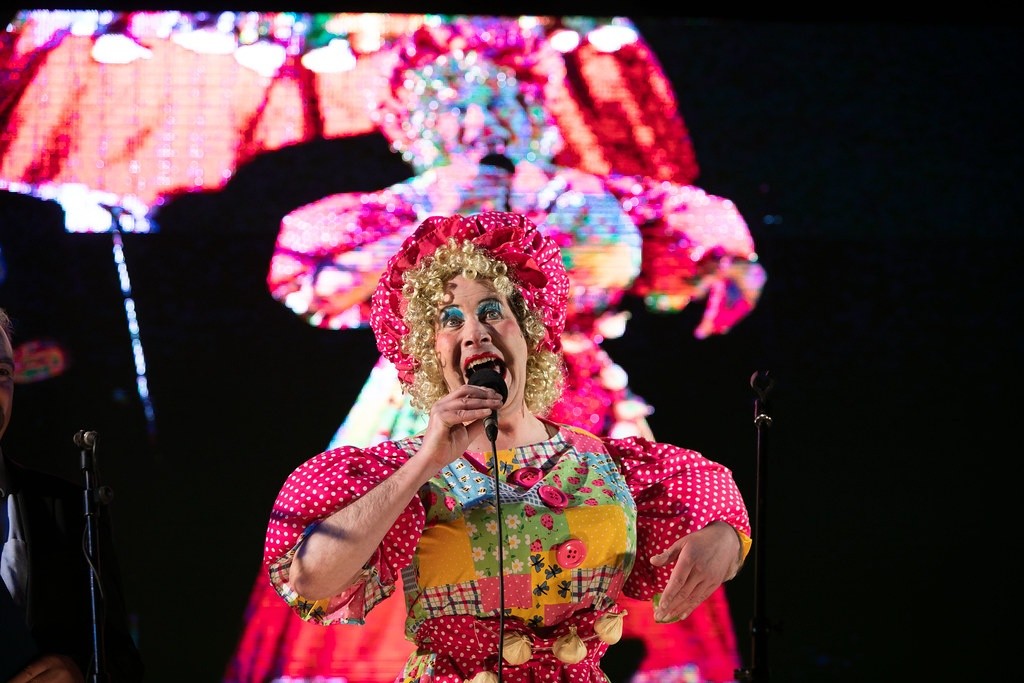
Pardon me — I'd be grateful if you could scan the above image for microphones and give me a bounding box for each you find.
[468,369,508,441]
[750,370,775,395]
[73,430,101,450]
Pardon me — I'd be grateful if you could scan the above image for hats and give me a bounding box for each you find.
[370,210,571,384]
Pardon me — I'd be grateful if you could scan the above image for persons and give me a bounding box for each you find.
[0,308,86,683]
[226,17,764,682]
